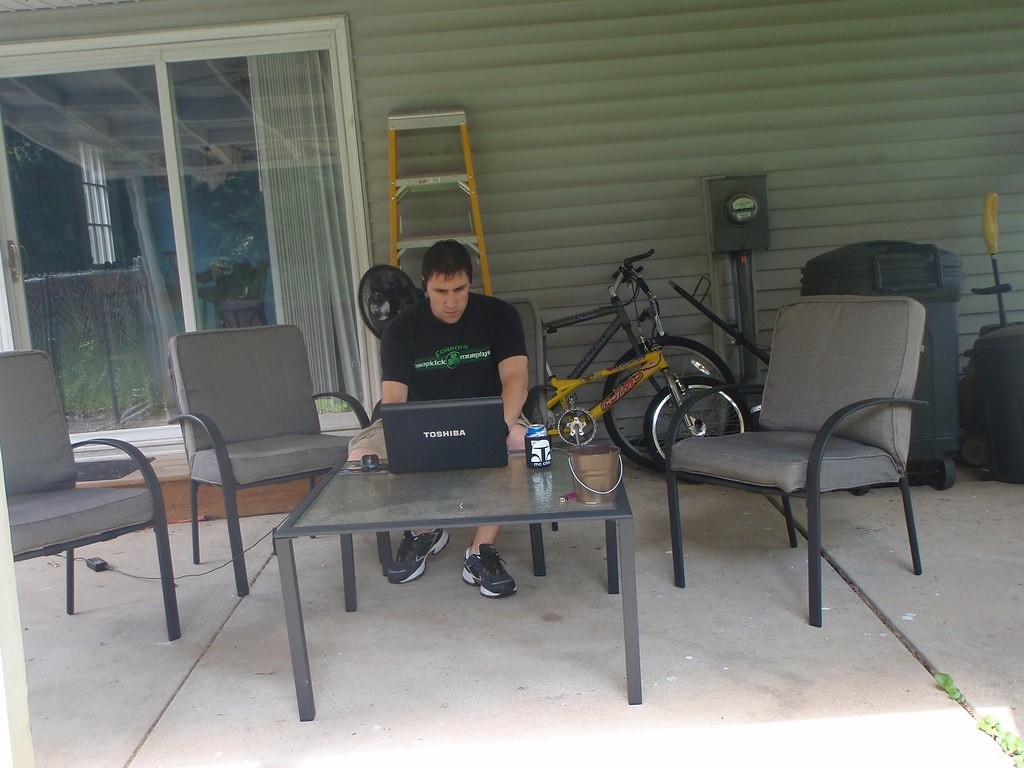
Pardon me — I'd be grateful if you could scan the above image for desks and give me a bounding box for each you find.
[273,447,644,722]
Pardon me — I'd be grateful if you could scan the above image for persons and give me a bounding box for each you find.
[346,239,530,599]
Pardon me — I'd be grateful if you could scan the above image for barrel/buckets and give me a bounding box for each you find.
[568,445,624,505]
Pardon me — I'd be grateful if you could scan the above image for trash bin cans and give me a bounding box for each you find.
[960,320,1024,484]
[800,241,965,496]
[219,300,274,328]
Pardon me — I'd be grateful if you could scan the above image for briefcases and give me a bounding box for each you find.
[800,241,961,303]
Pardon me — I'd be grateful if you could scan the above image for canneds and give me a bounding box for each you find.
[525,424,548,437]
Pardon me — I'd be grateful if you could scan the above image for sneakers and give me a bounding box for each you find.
[462,543,516,598]
[387,528,451,584]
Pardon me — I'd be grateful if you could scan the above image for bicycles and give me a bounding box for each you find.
[520,249,751,485]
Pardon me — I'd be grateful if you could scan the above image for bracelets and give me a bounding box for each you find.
[504,420,511,437]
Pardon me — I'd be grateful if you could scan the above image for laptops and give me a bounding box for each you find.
[380,396,508,474]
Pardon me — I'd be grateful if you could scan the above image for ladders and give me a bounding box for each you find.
[387,104,492,296]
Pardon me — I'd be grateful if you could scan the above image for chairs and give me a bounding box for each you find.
[220,306,271,327]
[0,350,181,642]
[167,325,384,597]
[370,297,553,575]
[668,297,932,628]
